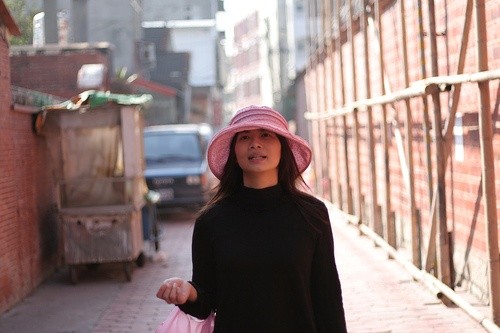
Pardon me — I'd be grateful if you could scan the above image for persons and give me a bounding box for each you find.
[155,106,348,333]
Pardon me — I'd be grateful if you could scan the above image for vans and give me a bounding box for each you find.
[141,123,219,215]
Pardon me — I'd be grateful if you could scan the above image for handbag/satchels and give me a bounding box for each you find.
[153,305,216,333]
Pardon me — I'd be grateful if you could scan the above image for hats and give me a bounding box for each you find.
[207,105,311,184]
